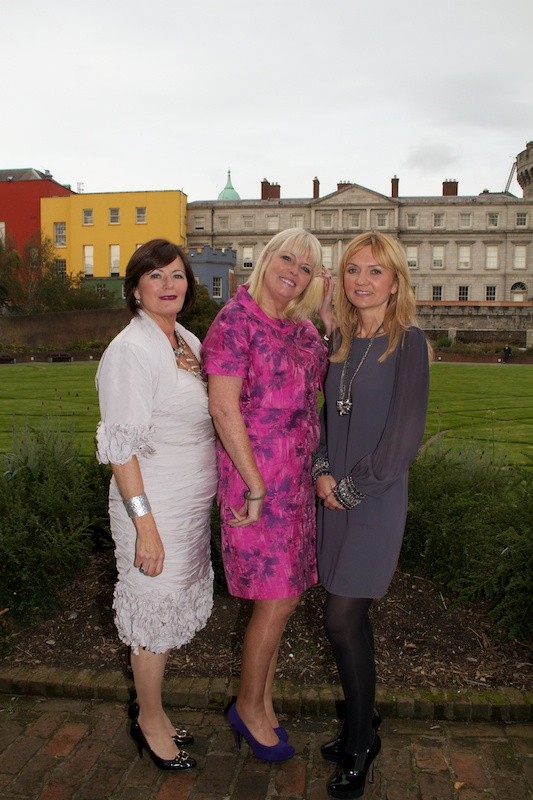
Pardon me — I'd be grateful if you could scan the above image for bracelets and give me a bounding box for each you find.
[123,493,151,518]
[245,491,267,501]
[324,335,329,341]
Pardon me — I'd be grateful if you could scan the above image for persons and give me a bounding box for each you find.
[94,239,219,770]
[503,343,511,362]
[200,228,338,764]
[311,230,430,800]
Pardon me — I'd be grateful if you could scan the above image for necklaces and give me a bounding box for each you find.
[171,328,200,377]
[336,322,383,417]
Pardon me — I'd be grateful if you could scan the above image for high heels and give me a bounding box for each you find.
[127,700,194,748]
[326,734,382,800]
[272,722,289,742]
[321,708,381,761]
[227,703,295,761]
[130,718,197,771]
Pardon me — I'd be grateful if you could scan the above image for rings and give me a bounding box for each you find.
[140,568,144,573]
